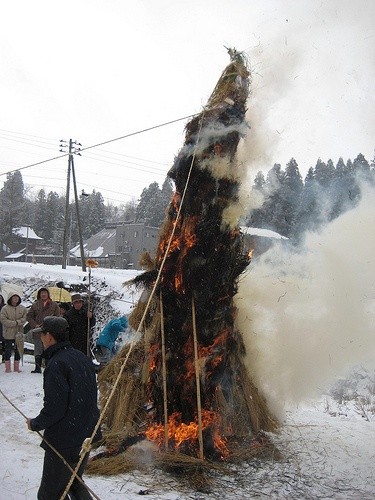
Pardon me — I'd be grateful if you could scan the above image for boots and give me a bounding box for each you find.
[14,360,22,373]
[31,355,43,373]
[5,360,11,373]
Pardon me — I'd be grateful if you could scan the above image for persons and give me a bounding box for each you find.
[27,317,102,500]
[63,294,96,354]
[0,291,27,372]
[0,294,7,363]
[27,287,61,373]
[96,316,129,377]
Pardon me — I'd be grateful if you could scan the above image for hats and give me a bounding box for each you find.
[71,295,84,304]
[32,315,70,334]
